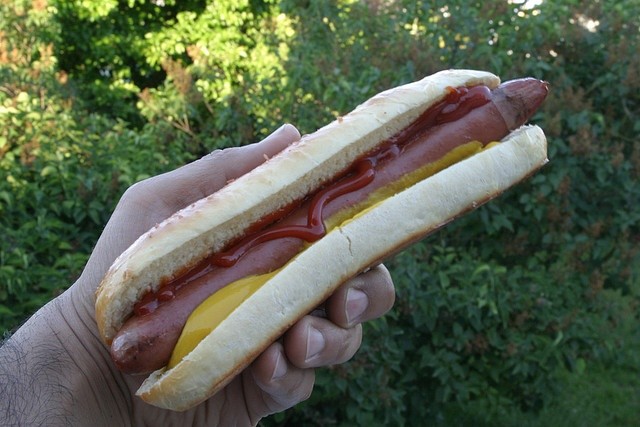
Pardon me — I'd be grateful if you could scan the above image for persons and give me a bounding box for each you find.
[0,121,396,426]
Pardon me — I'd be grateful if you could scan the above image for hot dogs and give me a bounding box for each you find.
[95,69,549,412]
